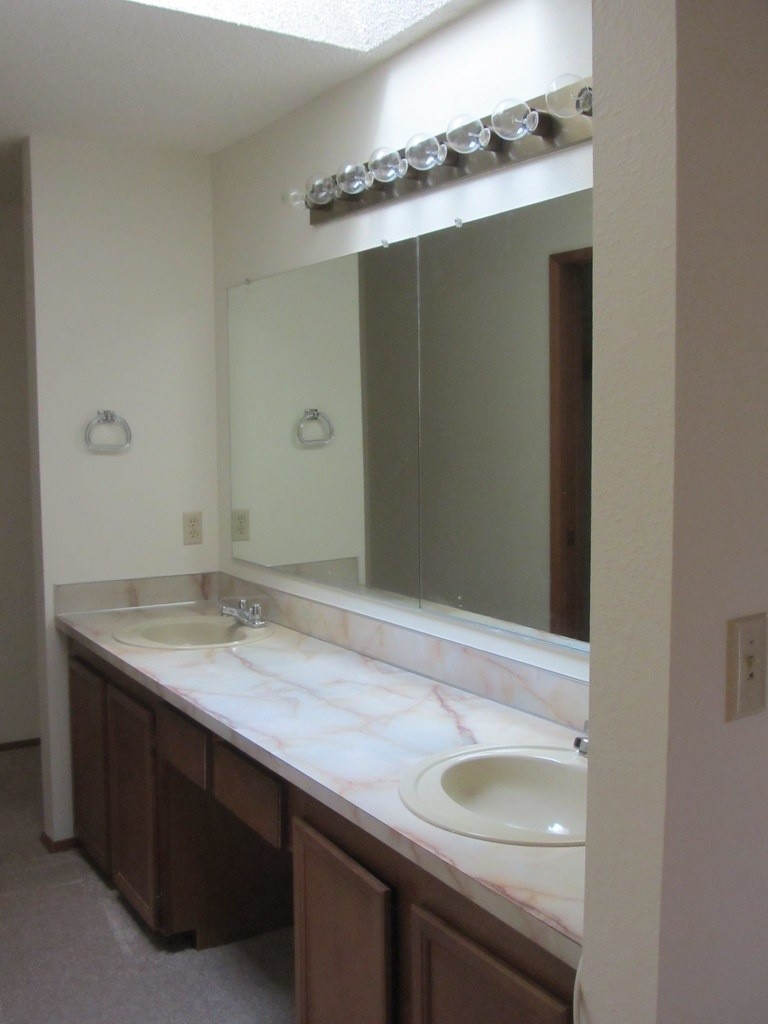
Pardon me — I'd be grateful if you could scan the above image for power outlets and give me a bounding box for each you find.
[183,511,203,545]
[230,509,249,542]
[726,613,768,721]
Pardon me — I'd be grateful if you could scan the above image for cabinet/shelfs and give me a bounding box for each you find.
[67,633,577,1024]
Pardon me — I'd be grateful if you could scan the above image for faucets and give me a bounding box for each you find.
[572,735,589,758]
[219,603,267,629]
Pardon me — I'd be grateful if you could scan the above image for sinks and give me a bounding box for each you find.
[397,744,586,850]
[111,615,275,652]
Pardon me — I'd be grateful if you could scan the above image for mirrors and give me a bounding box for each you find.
[228,188,592,654]
